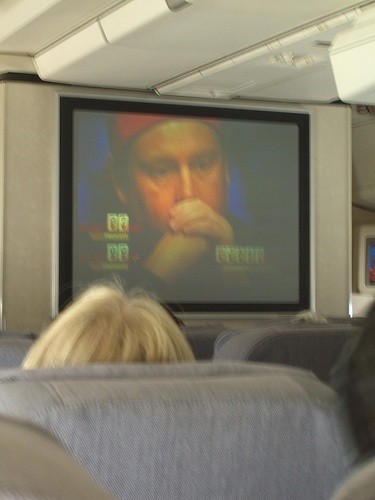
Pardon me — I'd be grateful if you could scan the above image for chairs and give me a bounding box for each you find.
[0,317,375,500]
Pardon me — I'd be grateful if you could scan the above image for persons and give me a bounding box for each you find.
[292,310,331,326]
[20,288,198,365]
[334,296,375,463]
[77,113,299,300]
